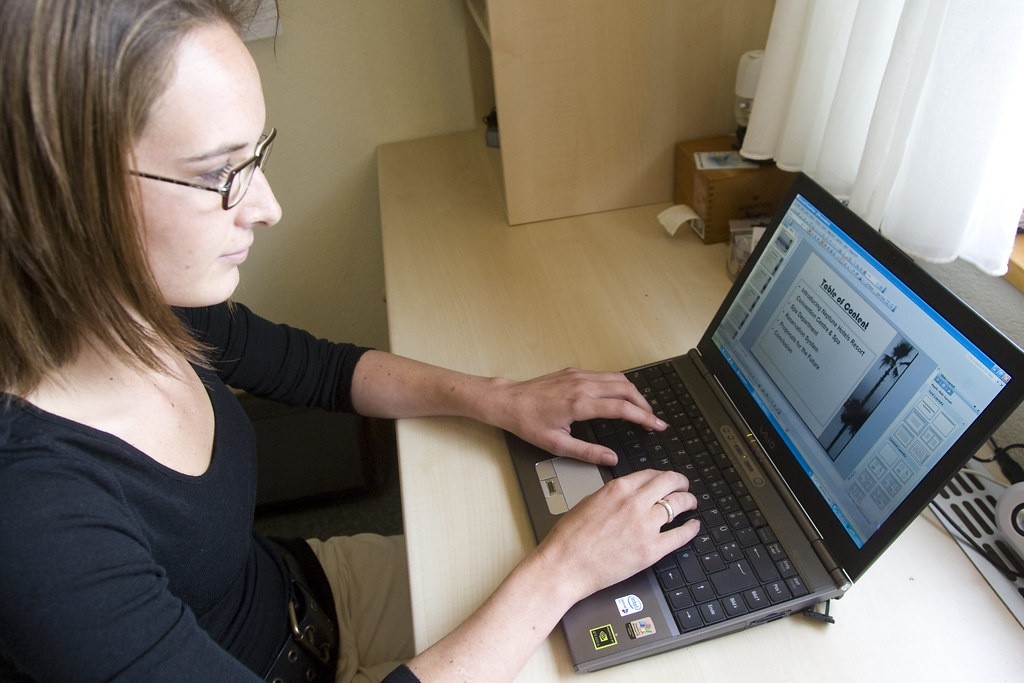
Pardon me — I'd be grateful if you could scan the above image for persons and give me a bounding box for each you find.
[0,1,704,683]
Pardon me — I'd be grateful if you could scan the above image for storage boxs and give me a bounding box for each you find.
[675,135,796,245]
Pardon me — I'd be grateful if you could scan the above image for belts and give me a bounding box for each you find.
[260,537,337,683]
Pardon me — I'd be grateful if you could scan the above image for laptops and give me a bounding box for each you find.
[500,168,1024,675]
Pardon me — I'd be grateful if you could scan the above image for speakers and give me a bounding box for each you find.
[994,482,1024,560]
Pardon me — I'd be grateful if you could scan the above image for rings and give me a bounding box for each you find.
[655,499,675,523]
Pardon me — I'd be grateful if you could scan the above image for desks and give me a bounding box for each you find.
[376,123,1024,683]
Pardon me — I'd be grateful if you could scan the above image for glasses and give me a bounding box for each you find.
[130,127,277,210]
